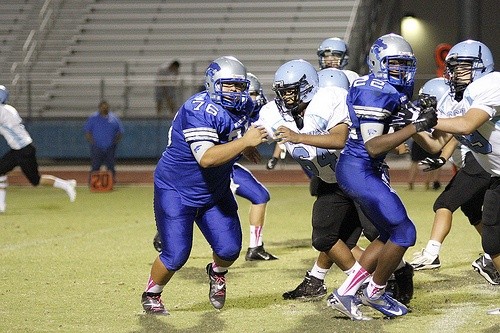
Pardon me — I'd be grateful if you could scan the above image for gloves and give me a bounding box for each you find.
[417,156,447,173]
[387,93,438,135]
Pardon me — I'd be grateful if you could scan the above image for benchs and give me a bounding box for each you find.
[0,0,378,120]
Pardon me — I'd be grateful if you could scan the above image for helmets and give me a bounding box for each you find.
[271,59,320,114]
[0,84,9,104]
[247,71,266,110]
[366,33,418,88]
[442,39,495,93]
[317,67,350,91]
[316,37,351,71]
[204,55,251,111]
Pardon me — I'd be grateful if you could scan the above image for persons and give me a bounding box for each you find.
[281,37,363,299]
[153,73,281,261]
[388,40,500,285]
[0,85,77,214]
[139,56,269,315]
[324,34,438,322]
[254,60,414,306]
[156,60,180,114]
[83,101,123,183]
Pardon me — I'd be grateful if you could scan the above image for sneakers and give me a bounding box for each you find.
[408,248,442,271]
[360,288,410,320]
[153,233,161,253]
[140,290,171,318]
[325,287,375,322]
[206,262,229,310]
[470,252,500,286]
[355,262,416,304]
[245,241,279,261]
[280,269,328,302]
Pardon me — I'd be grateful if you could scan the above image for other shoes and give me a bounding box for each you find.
[66,179,78,203]
[0,203,6,214]
